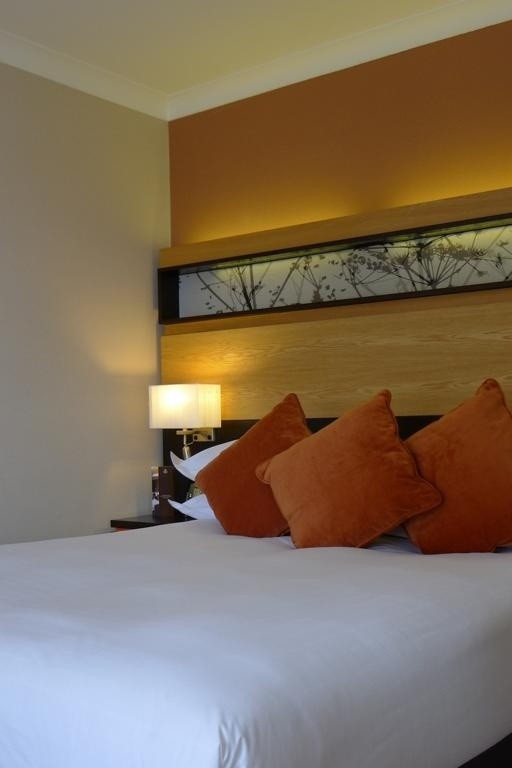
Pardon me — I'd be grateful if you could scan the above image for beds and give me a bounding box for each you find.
[0,414,512,768]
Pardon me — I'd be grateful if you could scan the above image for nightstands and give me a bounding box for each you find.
[109,516,197,532]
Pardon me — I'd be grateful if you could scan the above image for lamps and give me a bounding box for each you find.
[148,383,223,517]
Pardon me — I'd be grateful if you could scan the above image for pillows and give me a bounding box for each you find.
[253,389,444,550]
[194,391,315,538]
[169,440,240,481]
[404,379,512,555]
[167,493,217,519]
